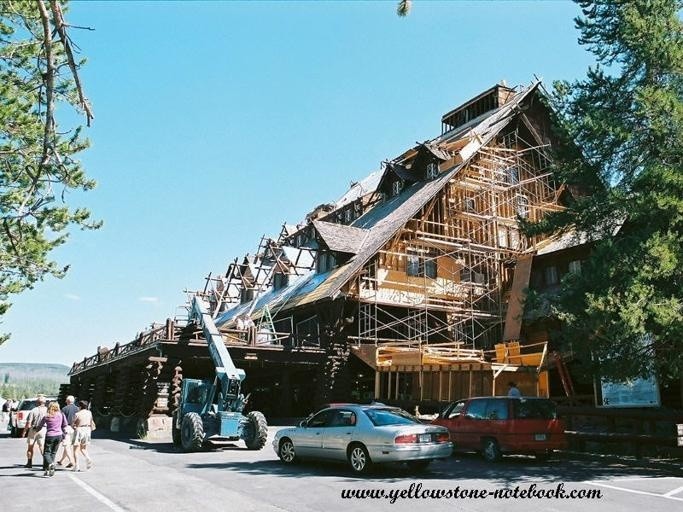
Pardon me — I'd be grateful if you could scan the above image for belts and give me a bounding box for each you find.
[80,424,91,428]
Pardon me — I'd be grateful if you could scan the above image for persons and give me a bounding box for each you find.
[0,398,12,432]
[69,400,97,472]
[507,382,522,396]
[9,397,19,410]
[56,394,79,468]
[34,401,68,477]
[21,394,47,471]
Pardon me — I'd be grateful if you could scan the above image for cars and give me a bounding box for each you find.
[11,395,58,438]
[272,396,567,473]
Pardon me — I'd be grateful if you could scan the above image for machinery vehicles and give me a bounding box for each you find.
[172,296,268,452]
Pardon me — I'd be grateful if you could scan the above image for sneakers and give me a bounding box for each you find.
[22,456,96,477]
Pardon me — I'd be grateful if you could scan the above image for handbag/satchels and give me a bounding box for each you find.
[90,418,96,432]
